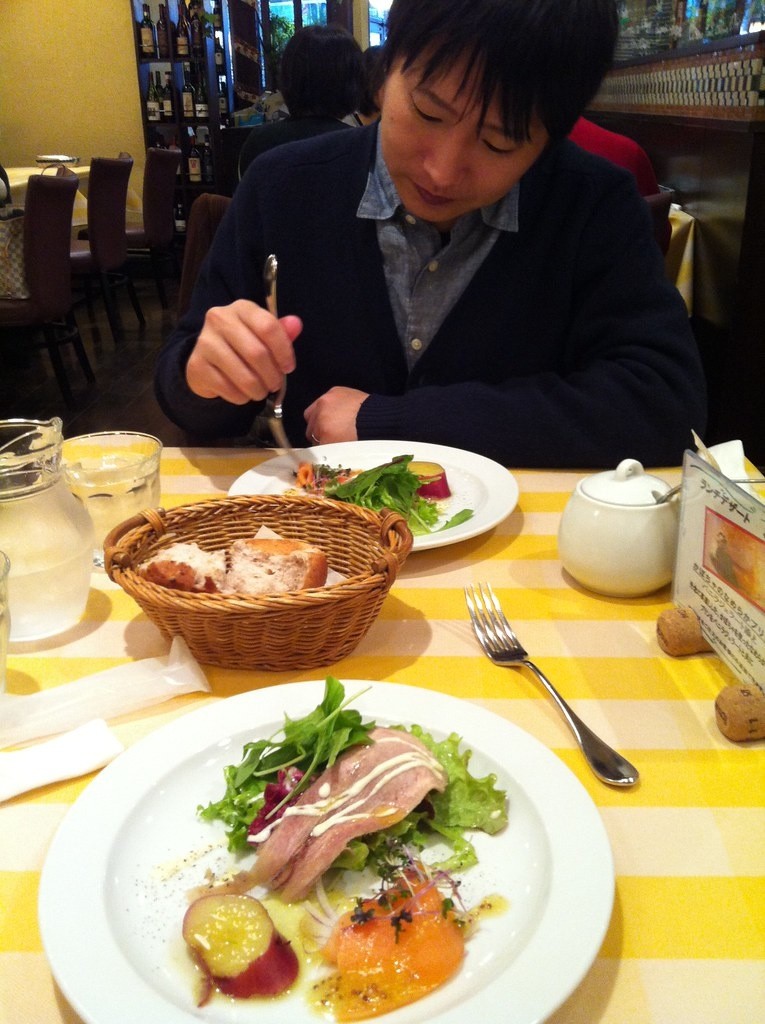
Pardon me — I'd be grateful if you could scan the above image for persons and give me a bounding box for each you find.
[568,116,672,258]
[342,46,384,128]
[238,24,368,181]
[153,0,708,467]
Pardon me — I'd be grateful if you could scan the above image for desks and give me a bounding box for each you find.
[0,163,91,227]
[662,203,696,320]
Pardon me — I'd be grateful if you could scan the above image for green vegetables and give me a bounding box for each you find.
[199,679,471,942]
[313,452,475,535]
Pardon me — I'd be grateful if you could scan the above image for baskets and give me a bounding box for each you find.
[102,495,413,669]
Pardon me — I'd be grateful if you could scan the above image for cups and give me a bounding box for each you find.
[60,431,162,568]
[558,458,681,599]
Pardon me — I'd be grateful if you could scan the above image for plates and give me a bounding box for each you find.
[40,678,619,1021]
[228,441,521,551]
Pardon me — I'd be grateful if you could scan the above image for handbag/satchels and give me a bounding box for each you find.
[0,162,70,300]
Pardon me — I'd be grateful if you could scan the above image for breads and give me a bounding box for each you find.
[136,537,328,594]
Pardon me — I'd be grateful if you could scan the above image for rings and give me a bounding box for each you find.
[312,434,320,442]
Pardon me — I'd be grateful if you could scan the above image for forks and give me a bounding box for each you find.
[465,584,639,786]
[263,254,304,467]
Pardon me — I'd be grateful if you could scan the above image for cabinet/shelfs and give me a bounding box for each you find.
[130,0,234,278]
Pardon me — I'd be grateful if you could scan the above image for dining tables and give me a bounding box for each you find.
[0,443,765,1024]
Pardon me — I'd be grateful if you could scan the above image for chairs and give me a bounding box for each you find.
[176,192,233,321]
[0,165,95,411]
[124,145,183,324]
[68,152,146,346]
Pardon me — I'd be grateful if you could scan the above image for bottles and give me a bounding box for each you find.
[135,0,228,232]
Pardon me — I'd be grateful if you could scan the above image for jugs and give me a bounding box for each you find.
[1,418,92,646]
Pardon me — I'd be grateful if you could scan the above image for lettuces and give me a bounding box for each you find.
[376,723,509,873]
